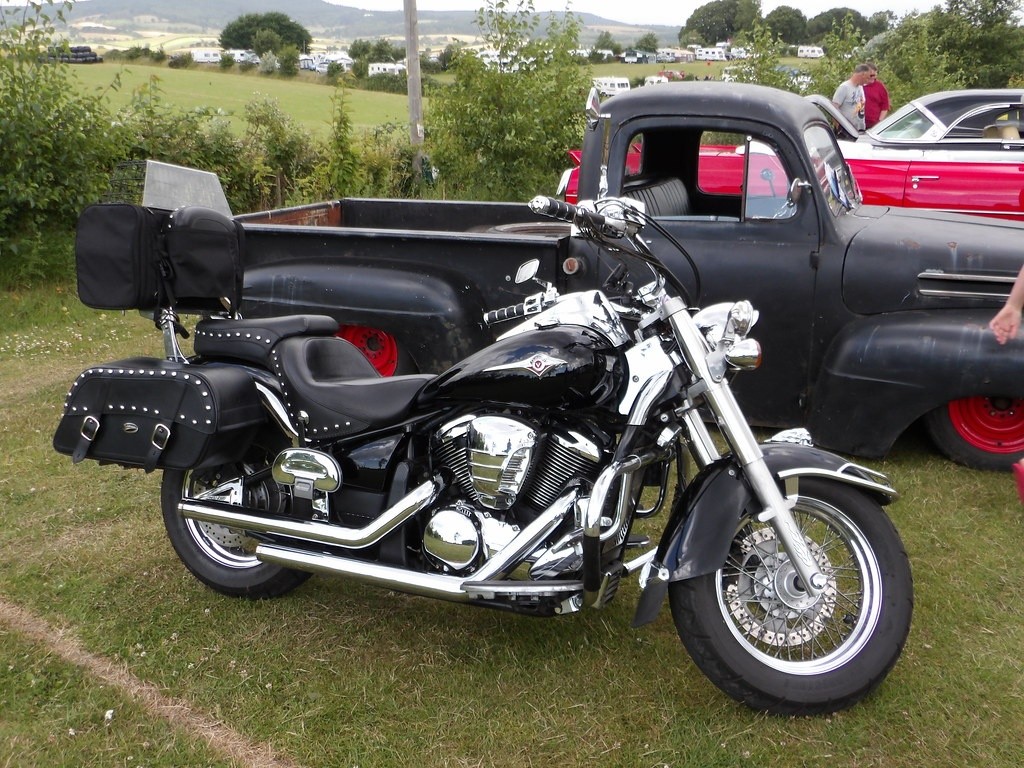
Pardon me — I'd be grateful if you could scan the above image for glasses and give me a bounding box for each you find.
[870,75,875,77]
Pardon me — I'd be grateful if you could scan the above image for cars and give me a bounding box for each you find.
[556,88,1023,221]
[191,41,825,96]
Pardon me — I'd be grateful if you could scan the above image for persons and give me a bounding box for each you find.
[695,74,716,81]
[988,262,1024,507]
[860,64,890,130]
[829,64,871,132]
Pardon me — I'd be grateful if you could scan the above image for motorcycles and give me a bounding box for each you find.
[45,200,914,715]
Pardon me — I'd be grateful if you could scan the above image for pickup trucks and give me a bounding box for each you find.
[230,78,1023,472]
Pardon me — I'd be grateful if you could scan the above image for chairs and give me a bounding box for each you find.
[984,123,1021,139]
[619,177,691,218]
